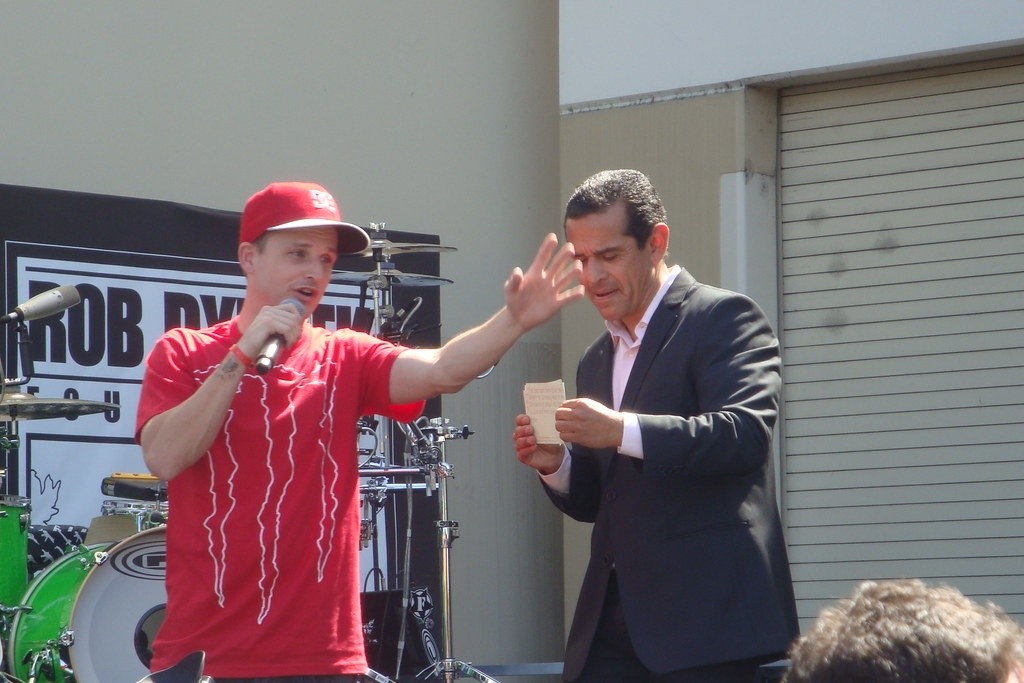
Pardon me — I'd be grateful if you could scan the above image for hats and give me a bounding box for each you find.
[239,182,371,256]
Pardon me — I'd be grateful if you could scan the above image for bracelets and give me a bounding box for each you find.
[229,343,255,369]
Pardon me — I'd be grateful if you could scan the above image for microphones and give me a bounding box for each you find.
[380,298,417,333]
[0,285,81,325]
[255,299,307,375]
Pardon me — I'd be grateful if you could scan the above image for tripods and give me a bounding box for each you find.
[407,422,503,683]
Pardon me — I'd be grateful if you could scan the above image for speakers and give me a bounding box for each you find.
[359,588,445,683]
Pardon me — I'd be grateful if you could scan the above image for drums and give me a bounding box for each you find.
[66,524,166,683]
[0,493,31,649]
[101,500,169,532]
[8,540,114,683]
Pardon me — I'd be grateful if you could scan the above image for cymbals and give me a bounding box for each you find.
[339,239,459,258]
[0,392,121,420]
[328,268,455,288]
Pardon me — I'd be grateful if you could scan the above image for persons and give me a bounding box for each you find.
[778,579,1024,683]
[512,166,802,682]
[135,179,588,683]
